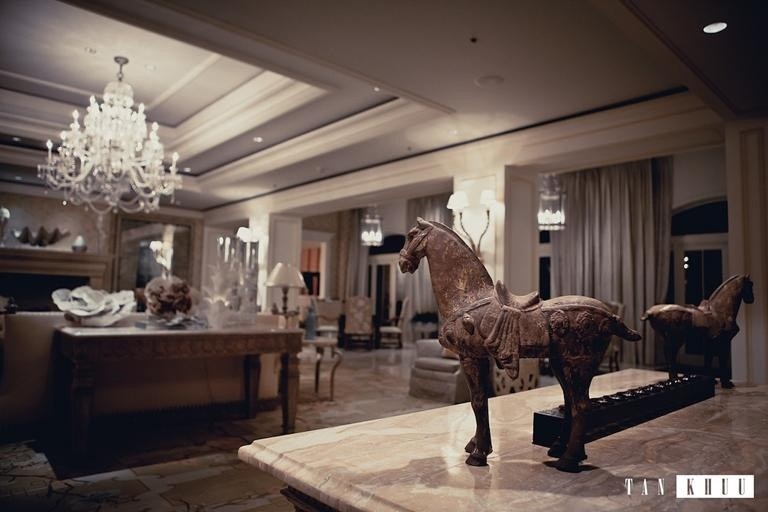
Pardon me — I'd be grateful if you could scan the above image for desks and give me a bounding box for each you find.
[237,369,768,512]
[54,319,302,453]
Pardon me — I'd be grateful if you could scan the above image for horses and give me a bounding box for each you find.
[640,271,755,390]
[396,214,642,473]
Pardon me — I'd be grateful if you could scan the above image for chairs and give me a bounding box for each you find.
[409,336,471,404]
[308,294,412,351]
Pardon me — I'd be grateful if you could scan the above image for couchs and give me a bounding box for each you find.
[1,308,281,426]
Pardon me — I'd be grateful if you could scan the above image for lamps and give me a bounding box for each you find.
[35,56,183,216]
[446,189,496,262]
[263,260,306,316]
